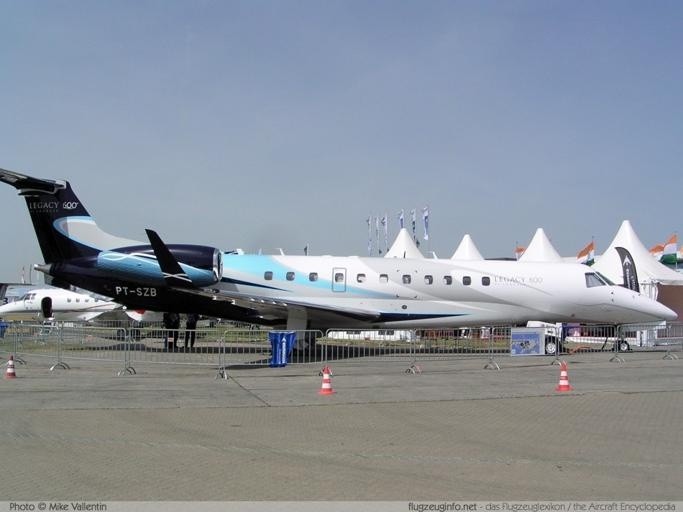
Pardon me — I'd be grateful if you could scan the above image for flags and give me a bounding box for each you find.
[373,216,381,254]
[399,210,403,227]
[382,215,387,254]
[410,208,415,244]
[675,246,682,263]
[421,204,428,239]
[576,241,594,267]
[649,234,676,264]
[365,215,372,255]
[514,246,526,260]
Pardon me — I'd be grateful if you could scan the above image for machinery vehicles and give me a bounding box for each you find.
[525,319,640,355]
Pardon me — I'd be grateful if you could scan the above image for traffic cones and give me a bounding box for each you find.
[2,354,17,380]
[316,363,337,396]
[553,359,575,393]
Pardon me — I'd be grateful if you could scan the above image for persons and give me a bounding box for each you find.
[163,311,180,348]
[183,313,197,349]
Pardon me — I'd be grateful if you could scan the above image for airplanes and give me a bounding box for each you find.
[0,167,682,356]
[0,286,163,343]
